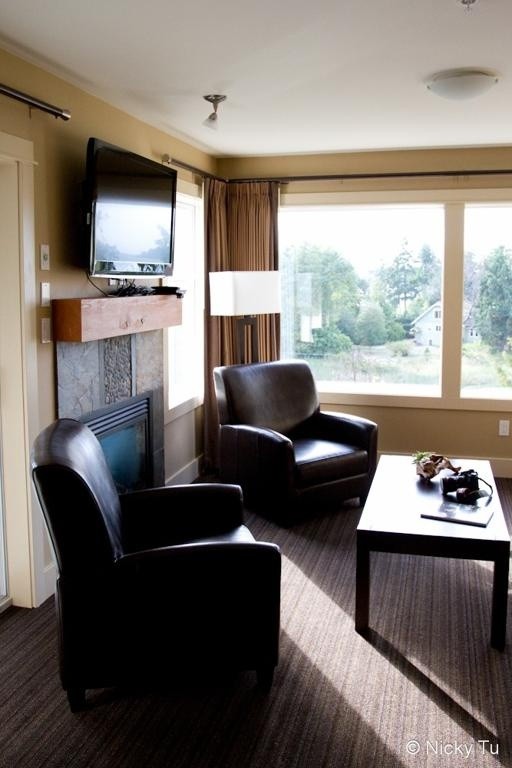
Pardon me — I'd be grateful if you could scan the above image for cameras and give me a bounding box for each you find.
[441,470,479,494]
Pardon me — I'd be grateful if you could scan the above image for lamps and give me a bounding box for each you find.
[207,268,283,365]
[418,68,501,106]
[198,93,229,130]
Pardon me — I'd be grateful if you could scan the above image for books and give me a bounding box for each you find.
[420,498,497,528]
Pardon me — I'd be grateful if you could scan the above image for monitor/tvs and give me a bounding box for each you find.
[86,137,178,279]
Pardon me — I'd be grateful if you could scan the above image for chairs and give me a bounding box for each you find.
[212,361,380,523]
[25,415,285,715]
